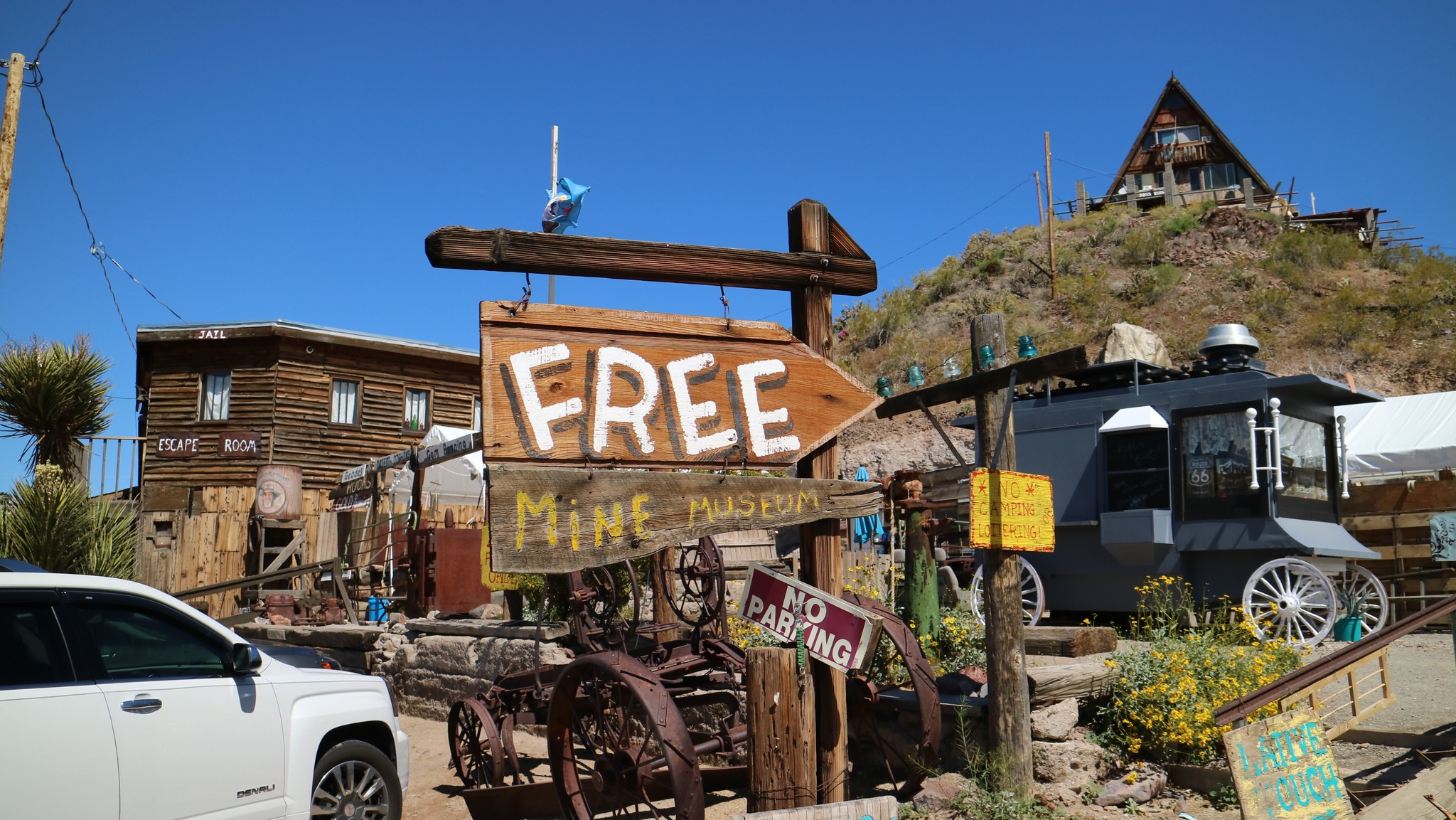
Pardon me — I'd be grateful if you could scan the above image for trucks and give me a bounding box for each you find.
[948,322,1389,648]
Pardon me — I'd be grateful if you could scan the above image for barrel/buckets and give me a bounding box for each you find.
[1334,616,1364,643]
[368,597,389,622]
[254,464,303,520]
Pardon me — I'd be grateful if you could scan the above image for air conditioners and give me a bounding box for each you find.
[1202,136,1211,143]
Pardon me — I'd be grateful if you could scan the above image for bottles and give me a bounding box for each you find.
[420,423,423,430]
[405,418,410,429]
[411,414,417,430]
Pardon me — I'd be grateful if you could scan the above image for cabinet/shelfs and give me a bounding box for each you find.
[407,528,492,617]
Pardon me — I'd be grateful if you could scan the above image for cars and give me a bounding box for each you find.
[0,570,411,820]
